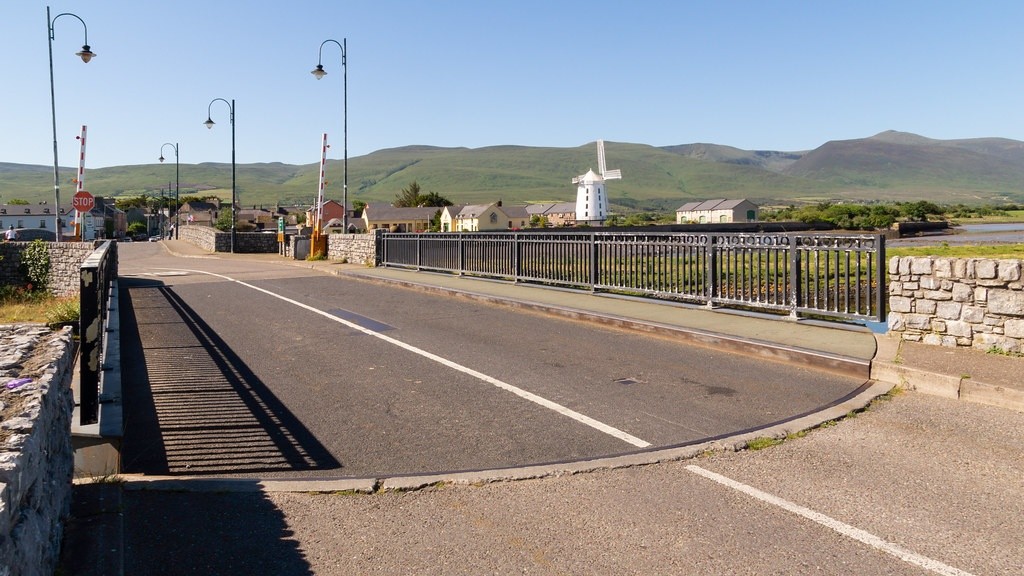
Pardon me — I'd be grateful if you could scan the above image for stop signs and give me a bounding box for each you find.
[72,190,95,212]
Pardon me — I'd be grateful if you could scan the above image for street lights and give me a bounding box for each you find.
[203,97,236,253]
[159,142,179,240]
[47,5,97,241]
[308,37,348,234]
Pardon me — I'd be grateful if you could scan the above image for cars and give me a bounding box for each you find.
[0,226,72,242]
[123,233,161,242]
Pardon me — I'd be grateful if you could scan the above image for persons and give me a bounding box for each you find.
[167,223,175,240]
[5,224,18,241]
